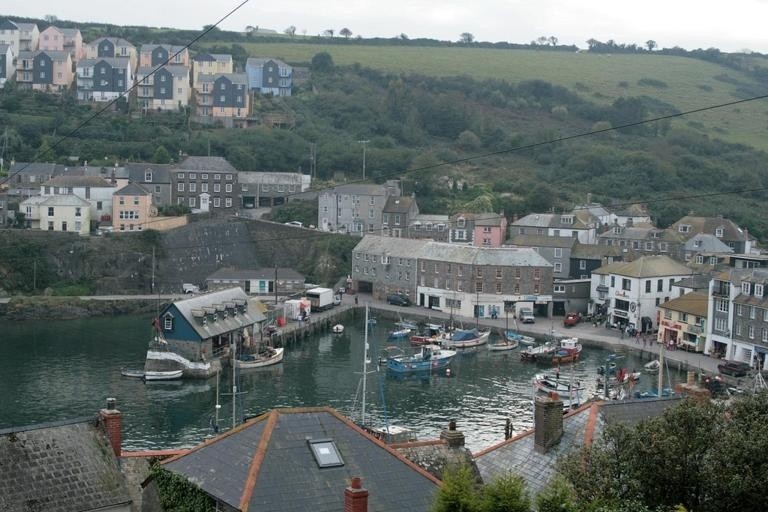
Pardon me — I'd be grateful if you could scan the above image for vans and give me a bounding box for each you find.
[386,292,411,306]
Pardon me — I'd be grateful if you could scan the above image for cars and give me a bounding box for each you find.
[519,308,535,324]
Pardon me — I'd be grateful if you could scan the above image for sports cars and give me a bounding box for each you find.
[564,312,581,327]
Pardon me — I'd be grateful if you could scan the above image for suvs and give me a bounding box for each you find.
[717,361,746,378]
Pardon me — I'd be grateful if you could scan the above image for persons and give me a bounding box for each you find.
[635,331,640,343]
[642,335,646,346]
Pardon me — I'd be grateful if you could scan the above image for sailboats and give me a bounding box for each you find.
[349,302,418,444]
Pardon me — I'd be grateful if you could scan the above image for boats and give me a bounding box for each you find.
[230,347,283,368]
[386,326,489,373]
[368,319,377,326]
[120,367,183,381]
[489,331,673,397]
[333,323,344,333]
